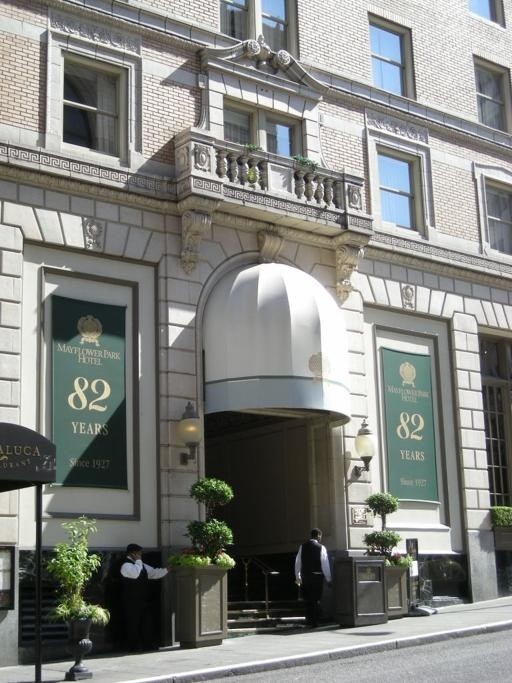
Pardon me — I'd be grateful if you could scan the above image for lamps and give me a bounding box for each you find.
[353,417,375,476]
[177,401,202,464]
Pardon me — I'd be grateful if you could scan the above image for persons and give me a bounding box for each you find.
[295,527,333,627]
[120,543,172,650]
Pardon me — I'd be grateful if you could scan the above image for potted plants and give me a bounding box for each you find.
[165,478,234,649]
[49,513,111,679]
[361,491,412,619]
